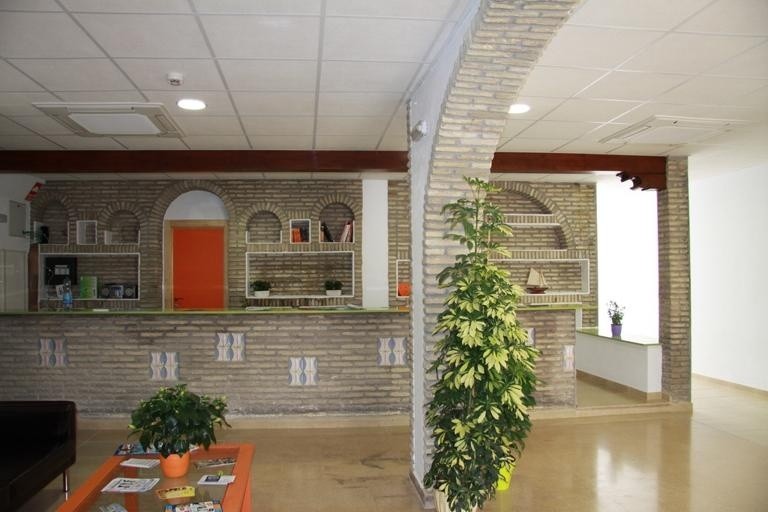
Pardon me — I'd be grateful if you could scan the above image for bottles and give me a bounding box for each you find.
[63,287,72,305]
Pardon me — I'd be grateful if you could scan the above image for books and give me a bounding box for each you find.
[100,443,236,512]
[291,219,352,243]
[78,275,98,300]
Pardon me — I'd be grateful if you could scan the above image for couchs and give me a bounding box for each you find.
[1,400,76,511]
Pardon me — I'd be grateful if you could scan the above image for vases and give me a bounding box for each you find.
[488,456,515,491]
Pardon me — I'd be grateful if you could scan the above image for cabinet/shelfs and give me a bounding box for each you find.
[484,181,591,298]
[34,193,147,301]
[237,193,356,299]
[0,249,30,313]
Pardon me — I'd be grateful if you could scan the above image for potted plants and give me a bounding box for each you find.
[129,382,233,479]
[250,280,271,297]
[608,301,626,337]
[324,280,343,296]
[419,171,543,512]
